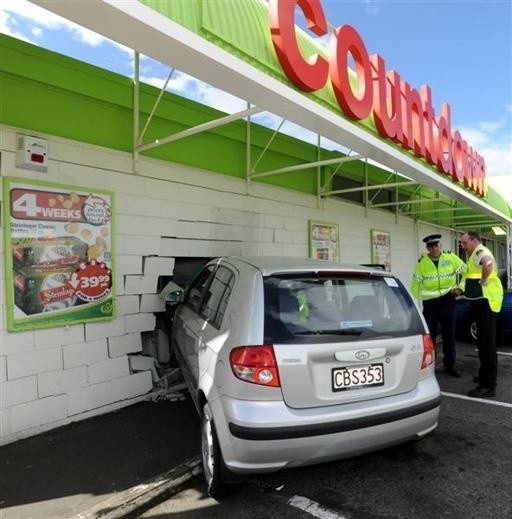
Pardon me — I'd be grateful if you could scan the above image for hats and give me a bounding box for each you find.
[422,233,442,245]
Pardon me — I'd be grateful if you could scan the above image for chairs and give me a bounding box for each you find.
[343,295,384,326]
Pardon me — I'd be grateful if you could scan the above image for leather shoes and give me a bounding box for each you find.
[468,386,496,397]
[471,375,479,381]
[445,367,461,378]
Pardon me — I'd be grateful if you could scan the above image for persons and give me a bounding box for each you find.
[459,231,503,397]
[412,233,466,377]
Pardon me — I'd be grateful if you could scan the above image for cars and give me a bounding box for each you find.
[168,252,445,499]
[454,291,512,346]
